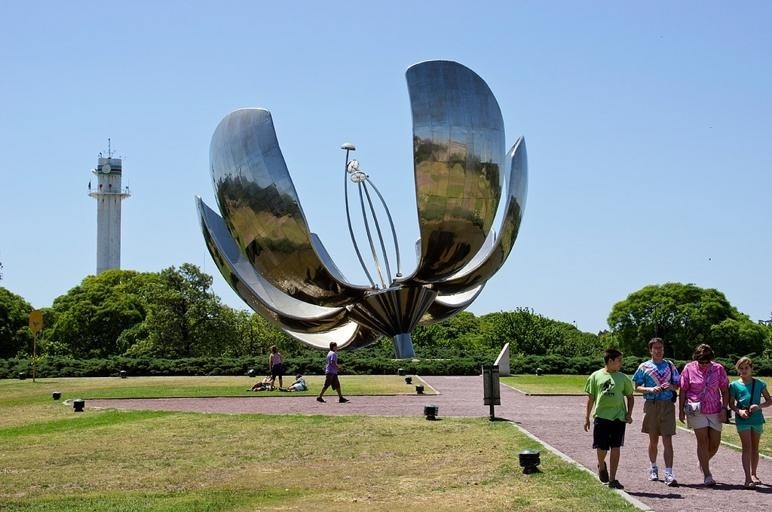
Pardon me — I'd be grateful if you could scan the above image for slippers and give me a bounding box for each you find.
[745,480,754,487]
[751,477,761,484]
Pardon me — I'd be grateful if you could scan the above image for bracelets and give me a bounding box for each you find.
[721,406,726,409]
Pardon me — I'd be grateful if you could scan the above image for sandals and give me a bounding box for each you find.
[698,459,716,485]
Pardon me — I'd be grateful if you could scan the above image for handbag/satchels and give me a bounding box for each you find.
[735,380,756,419]
[666,359,677,403]
[684,364,712,415]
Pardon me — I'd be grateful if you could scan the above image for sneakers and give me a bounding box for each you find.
[338,399,349,403]
[597,461,609,483]
[317,397,326,403]
[608,479,624,490]
[663,472,678,486]
[648,466,659,480]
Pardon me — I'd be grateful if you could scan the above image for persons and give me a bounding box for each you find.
[247,377,272,392]
[632,337,680,486]
[729,357,772,488]
[679,344,729,485]
[288,373,305,392]
[317,342,350,403]
[269,346,283,391]
[584,348,634,489]
